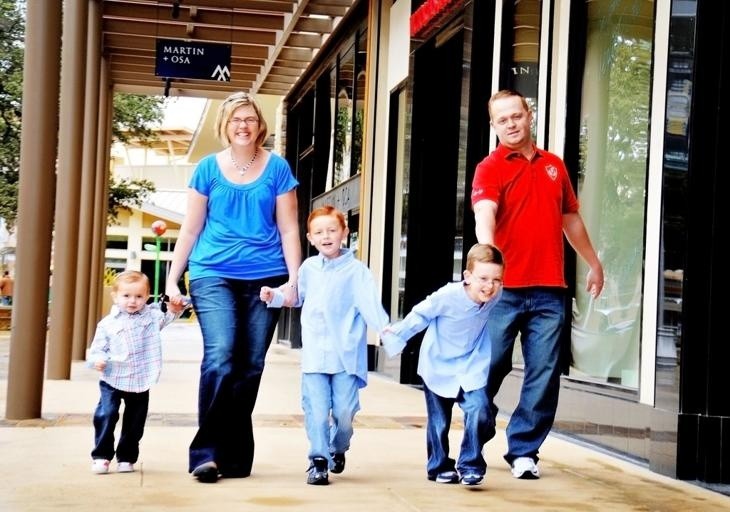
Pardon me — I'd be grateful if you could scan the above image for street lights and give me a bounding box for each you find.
[149,219,167,305]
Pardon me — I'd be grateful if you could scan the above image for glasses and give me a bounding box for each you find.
[227,117,260,125]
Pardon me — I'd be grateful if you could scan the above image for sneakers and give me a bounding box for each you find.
[511,456,540,478]
[306,456,328,484]
[193,460,220,483]
[117,461,134,471]
[459,472,483,485]
[90,458,110,474]
[436,469,458,484]
[328,453,345,473]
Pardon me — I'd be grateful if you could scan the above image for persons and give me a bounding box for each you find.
[470,88,605,480]
[260,206,392,485]
[89,269,184,474]
[0,271,14,307]
[164,91,304,483]
[381,243,504,487]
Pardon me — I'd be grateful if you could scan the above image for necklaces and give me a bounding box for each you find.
[230,146,258,178]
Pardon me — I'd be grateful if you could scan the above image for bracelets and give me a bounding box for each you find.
[285,282,298,291]
